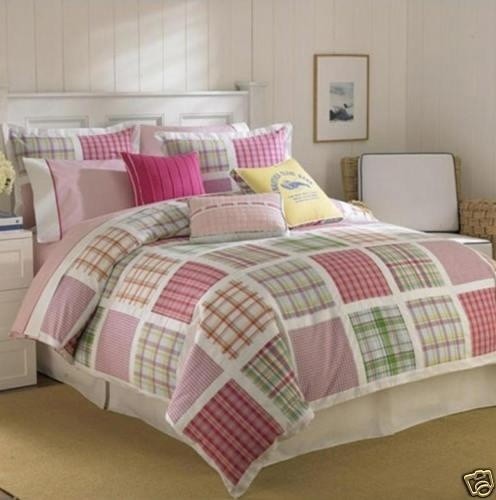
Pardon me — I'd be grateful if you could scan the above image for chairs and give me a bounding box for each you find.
[339,152,496,265]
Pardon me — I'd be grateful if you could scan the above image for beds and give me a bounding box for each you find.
[0,81,496,500]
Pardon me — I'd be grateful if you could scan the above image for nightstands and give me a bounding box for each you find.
[0,231,39,390]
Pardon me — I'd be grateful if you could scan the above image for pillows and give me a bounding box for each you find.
[2,123,142,230]
[19,156,133,244]
[151,123,292,198]
[120,150,204,209]
[187,192,287,243]
[230,158,343,233]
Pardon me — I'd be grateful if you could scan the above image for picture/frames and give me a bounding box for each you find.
[315,54,369,140]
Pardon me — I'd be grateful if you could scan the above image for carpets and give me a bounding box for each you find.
[0,381,496,500]
